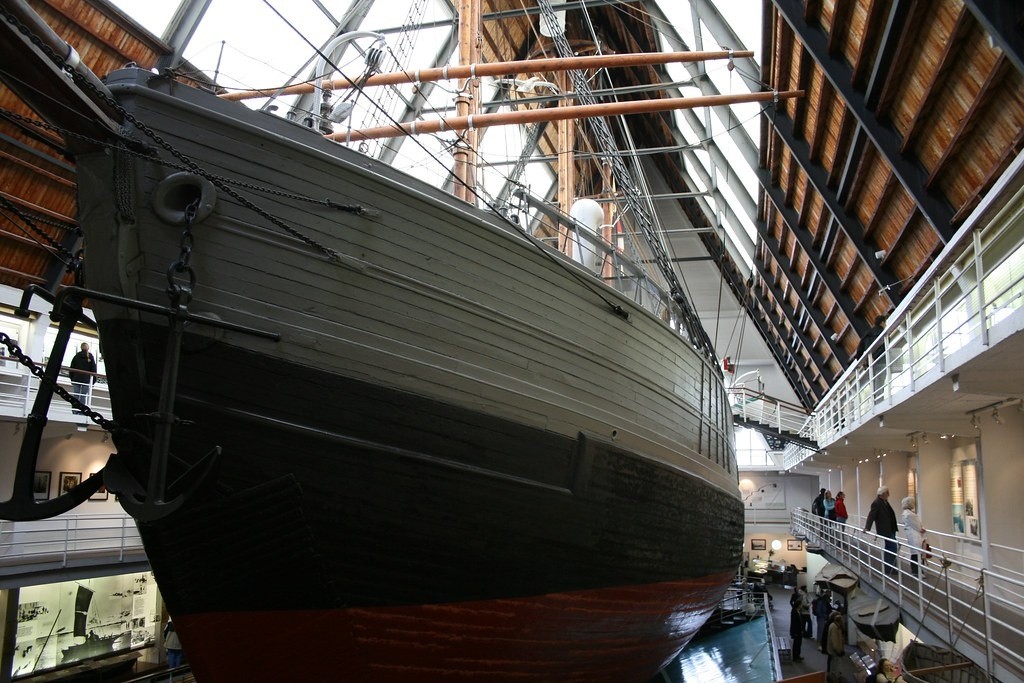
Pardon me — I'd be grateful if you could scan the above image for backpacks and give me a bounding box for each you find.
[811,597,823,615]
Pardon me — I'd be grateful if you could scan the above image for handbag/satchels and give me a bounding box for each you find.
[921,540,932,559]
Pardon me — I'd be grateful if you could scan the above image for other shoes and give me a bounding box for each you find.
[793,656,804,663]
[912,574,926,579]
[809,637,815,639]
[817,645,822,651]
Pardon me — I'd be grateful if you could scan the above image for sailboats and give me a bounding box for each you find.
[0,0,750,683]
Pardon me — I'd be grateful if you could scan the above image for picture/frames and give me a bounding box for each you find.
[787,539,802,551]
[89,473,108,500]
[34,471,51,501]
[58,472,82,498]
[751,539,766,550]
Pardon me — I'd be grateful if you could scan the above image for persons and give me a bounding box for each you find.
[874,658,907,683]
[901,496,927,578]
[790,600,805,663]
[812,488,826,537]
[164,621,183,668]
[863,485,898,575]
[790,585,846,673]
[834,492,848,547]
[823,490,836,539]
[67,342,98,415]
[866,315,890,404]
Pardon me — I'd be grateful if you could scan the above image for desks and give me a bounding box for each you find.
[767,569,807,589]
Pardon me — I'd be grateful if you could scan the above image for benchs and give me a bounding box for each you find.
[776,636,791,661]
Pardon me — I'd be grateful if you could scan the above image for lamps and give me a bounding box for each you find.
[970,413,982,430]
[830,333,838,340]
[875,250,886,259]
[922,431,929,443]
[1017,399,1024,412]
[910,435,917,447]
[992,406,1004,425]
